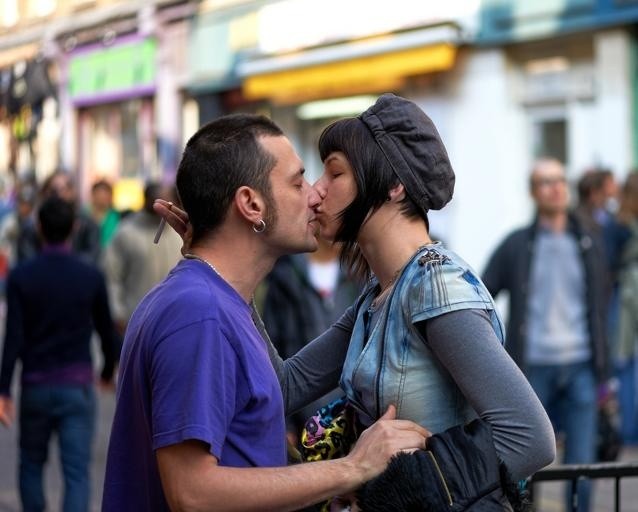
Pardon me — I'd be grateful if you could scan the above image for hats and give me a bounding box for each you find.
[359,92,455,213]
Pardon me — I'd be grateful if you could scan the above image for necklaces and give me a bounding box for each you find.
[185,253,224,280]
[375,268,402,301]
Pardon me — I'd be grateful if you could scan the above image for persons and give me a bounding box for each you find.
[2,165,184,512]
[480,159,638,512]
[101,113,434,512]
[153,94,557,512]
[254,245,375,446]
